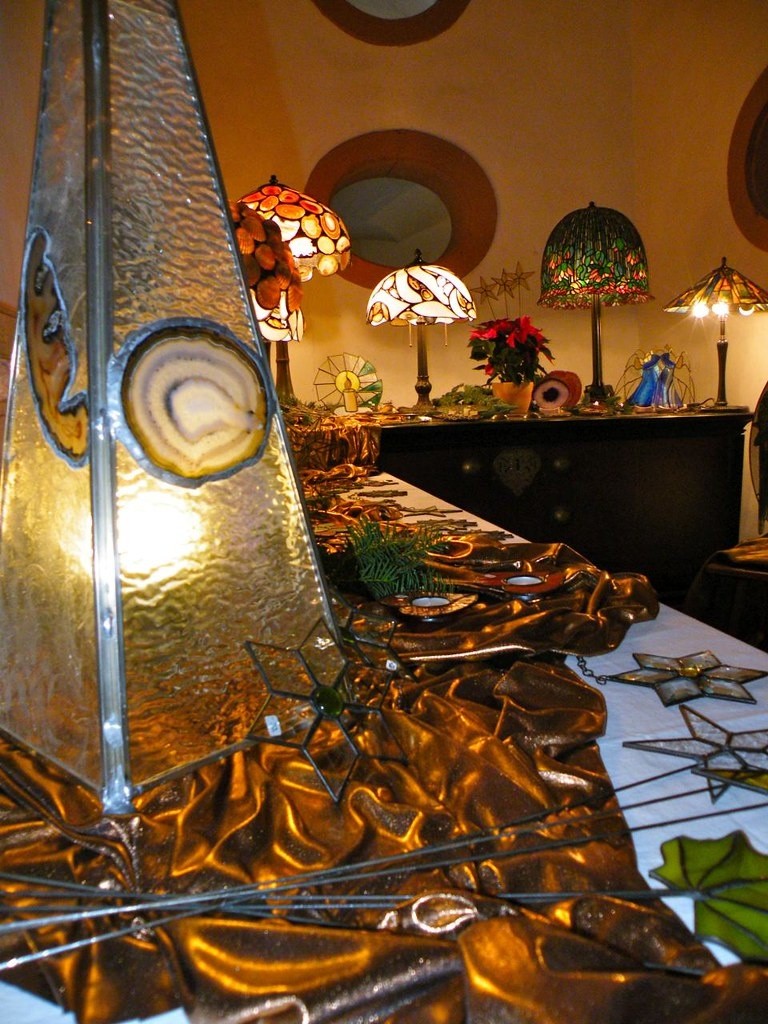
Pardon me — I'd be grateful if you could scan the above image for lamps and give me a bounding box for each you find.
[0,0,341,816]
[537,202,655,407]
[663,256,768,414]
[364,247,478,416]
[226,173,353,412]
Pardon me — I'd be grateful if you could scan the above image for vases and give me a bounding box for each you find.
[490,382,534,413]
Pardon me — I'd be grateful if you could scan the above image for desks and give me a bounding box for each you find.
[0,458,768,1024]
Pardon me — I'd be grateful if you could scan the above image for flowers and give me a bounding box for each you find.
[466,318,554,386]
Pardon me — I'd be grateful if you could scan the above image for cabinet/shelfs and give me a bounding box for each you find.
[338,412,755,567]
